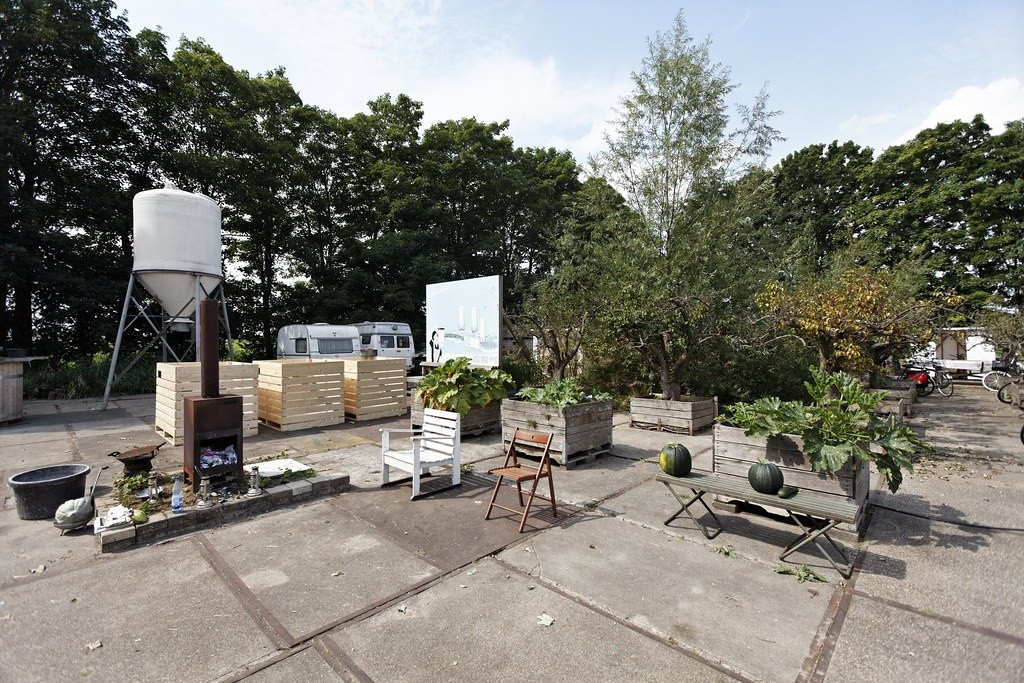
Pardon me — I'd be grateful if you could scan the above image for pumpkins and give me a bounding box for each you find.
[748,458,783,494]
[659,443,691,477]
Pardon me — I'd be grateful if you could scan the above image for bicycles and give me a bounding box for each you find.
[909,358,954,397]
[996,374,1024,405]
[884,360,935,398]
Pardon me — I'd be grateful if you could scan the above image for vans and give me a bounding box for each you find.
[347,321,415,374]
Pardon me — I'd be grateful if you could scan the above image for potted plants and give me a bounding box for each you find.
[411,356,516,437]
[713,364,933,531]
[500,379,613,466]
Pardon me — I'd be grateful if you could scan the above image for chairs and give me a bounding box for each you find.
[485,426,556,534]
[378,407,463,501]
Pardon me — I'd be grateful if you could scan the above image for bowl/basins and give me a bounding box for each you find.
[8,463,91,520]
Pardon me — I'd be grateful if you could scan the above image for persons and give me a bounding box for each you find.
[429,331,442,363]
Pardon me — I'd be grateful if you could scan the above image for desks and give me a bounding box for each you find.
[656,471,860,579]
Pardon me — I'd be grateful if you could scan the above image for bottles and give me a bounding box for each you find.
[171,476,183,514]
